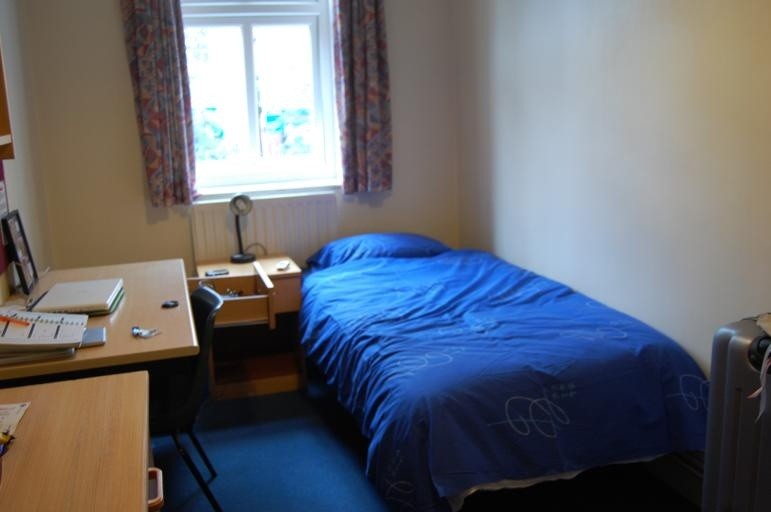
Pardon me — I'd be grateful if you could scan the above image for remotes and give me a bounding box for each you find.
[276,260,290,270]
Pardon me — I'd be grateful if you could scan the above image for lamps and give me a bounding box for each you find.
[226,191,257,264]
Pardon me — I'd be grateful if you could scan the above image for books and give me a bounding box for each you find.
[0,276,126,367]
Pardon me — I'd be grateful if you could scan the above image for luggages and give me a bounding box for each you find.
[701,314,771,512]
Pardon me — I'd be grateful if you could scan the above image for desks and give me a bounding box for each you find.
[2,367,163,511]
[1,257,205,380]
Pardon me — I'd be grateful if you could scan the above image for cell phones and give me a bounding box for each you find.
[205,268,229,276]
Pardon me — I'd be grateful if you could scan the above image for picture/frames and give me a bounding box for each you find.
[0,204,38,298]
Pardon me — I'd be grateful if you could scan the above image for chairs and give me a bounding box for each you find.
[149,282,226,511]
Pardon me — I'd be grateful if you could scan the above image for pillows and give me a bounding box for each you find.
[307,232,449,263]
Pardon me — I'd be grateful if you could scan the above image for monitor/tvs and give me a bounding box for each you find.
[31,278,124,314]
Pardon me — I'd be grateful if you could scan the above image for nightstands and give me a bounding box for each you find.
[197,255,302,400]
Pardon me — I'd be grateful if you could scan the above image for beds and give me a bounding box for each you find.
[301,251,706,511]
